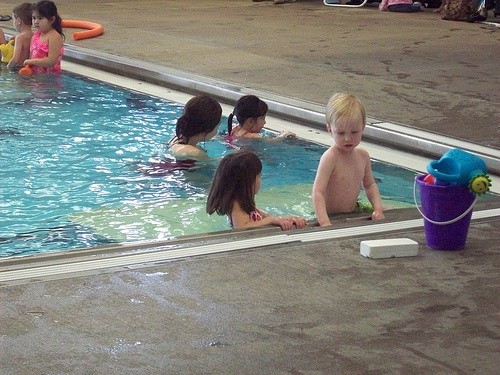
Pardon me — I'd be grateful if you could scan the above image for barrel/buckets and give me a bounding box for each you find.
[414,174,478,249]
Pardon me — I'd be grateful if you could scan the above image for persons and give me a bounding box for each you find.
[220,95,296,152]
[205,151,307,231]
[23,1,66,74]
[0,26,14,48]
[312,92,387,226]
[6,2,35,70]
[136,96,224,176]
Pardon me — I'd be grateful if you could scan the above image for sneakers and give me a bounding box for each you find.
[414,2,425,11]
